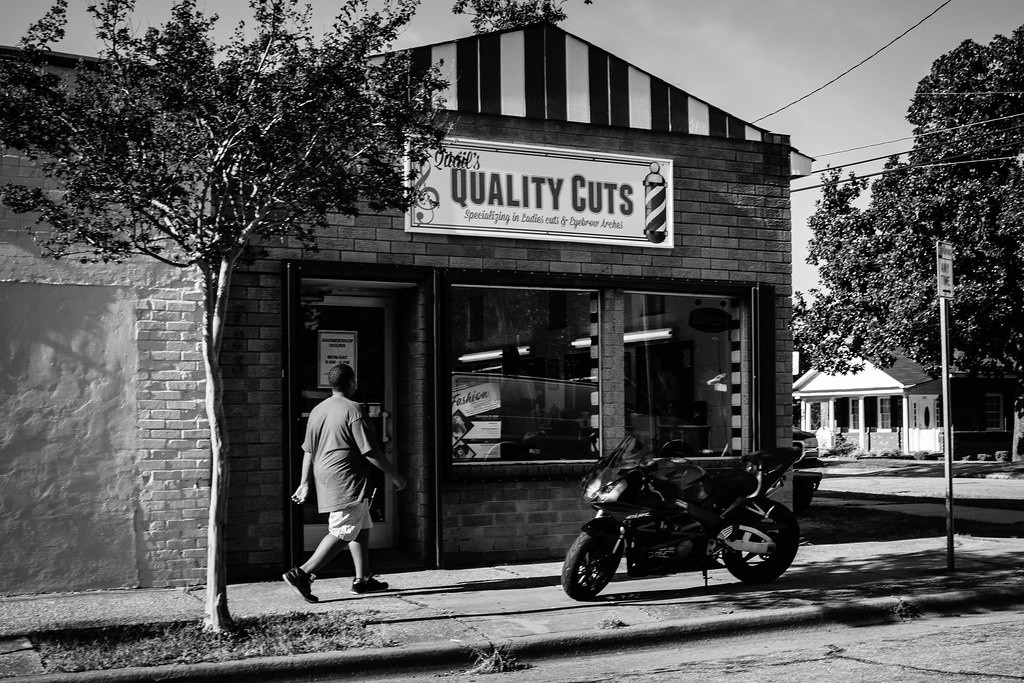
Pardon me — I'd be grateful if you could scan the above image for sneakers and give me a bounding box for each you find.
[350,572,389,595]
[282,566,319,603]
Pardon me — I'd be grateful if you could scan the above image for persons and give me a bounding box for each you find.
[282,364,406,603]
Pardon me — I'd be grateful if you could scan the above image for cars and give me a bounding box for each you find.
[520,419,591,451]
[300,393,389,525]
[793,426,819,465]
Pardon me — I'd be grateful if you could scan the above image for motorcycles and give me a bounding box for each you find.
[558,437,805,605]
